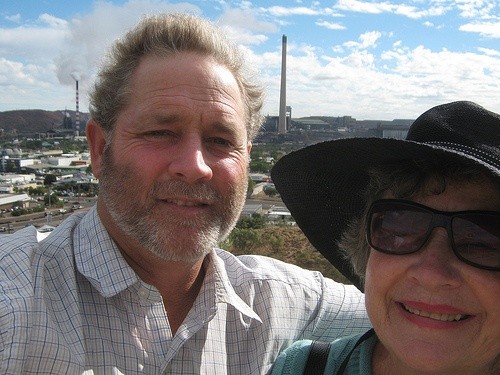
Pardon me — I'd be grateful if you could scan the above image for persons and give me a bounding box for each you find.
[0,11,374,375]
[262,101,500,375]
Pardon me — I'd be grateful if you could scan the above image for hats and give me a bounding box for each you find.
[270,101,500,294]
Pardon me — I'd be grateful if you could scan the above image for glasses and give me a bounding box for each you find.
[364,199,500,271]
